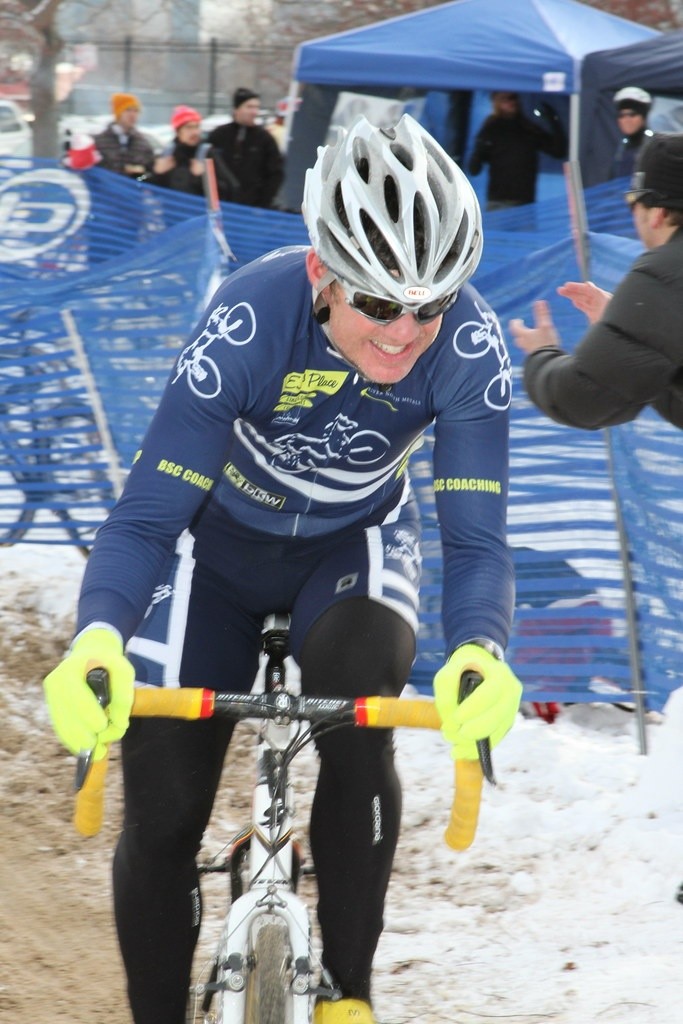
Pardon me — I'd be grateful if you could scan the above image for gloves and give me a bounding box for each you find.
[42,631,136,759]
[433,645,522,760]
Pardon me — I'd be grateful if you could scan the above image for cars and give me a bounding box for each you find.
[59,111,278,160]
[0,100,31,157]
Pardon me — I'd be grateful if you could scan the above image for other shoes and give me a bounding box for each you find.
[312,999,374,1024]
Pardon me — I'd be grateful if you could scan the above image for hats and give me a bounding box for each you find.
[172,106,200,130]
[234,88,261,110]
[112,94,139,116]
[631,133,683,206]
[613,87,653,115]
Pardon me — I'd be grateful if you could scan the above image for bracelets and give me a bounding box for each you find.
[447,638,504,663]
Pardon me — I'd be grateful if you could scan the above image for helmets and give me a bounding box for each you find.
[302,112,484,308]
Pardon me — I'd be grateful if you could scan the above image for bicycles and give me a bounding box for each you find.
[71,611,498,1024]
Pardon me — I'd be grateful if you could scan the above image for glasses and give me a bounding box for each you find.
[333,277,457,325]
[616,112,638,117]
[623,188,669,204]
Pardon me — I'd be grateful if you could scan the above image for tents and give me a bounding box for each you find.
[270,0,683,160]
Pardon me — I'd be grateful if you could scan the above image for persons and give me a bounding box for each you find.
[91,93,156,186]
[205,87,285,209]
[154,105,233,204]
[44,113,522,1024]
[465,89,568,212]
[509,133,683,430]
[608,87,653,179]
